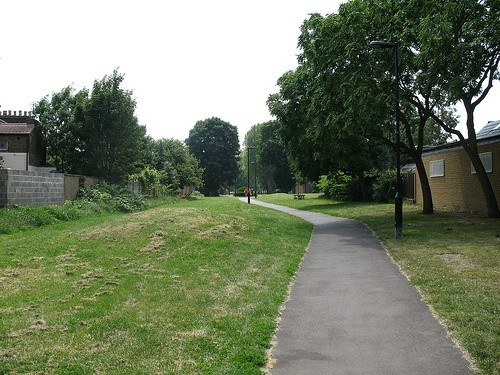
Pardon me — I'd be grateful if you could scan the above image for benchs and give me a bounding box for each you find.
[293,194,305,199]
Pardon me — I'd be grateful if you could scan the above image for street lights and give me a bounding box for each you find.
[371,38,403,240]
[247,147,256,204]
